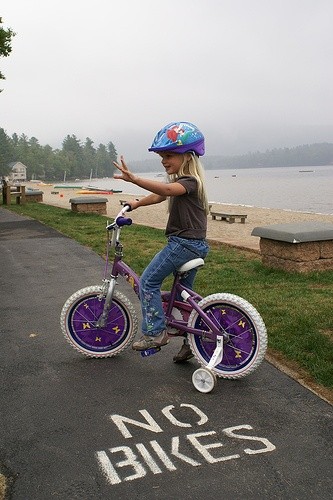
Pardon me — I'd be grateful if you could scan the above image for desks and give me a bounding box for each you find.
[3,185,25,205]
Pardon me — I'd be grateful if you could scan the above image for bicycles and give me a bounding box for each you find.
[60,205,267,394]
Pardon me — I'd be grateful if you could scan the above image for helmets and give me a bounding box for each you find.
[148,121,205,156]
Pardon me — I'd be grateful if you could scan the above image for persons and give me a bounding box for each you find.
[112,121,211,362]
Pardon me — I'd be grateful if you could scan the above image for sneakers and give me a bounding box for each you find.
[132,329,170,351]
[173,339,194,363]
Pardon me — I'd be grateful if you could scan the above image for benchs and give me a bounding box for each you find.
[210,212,248,224]
